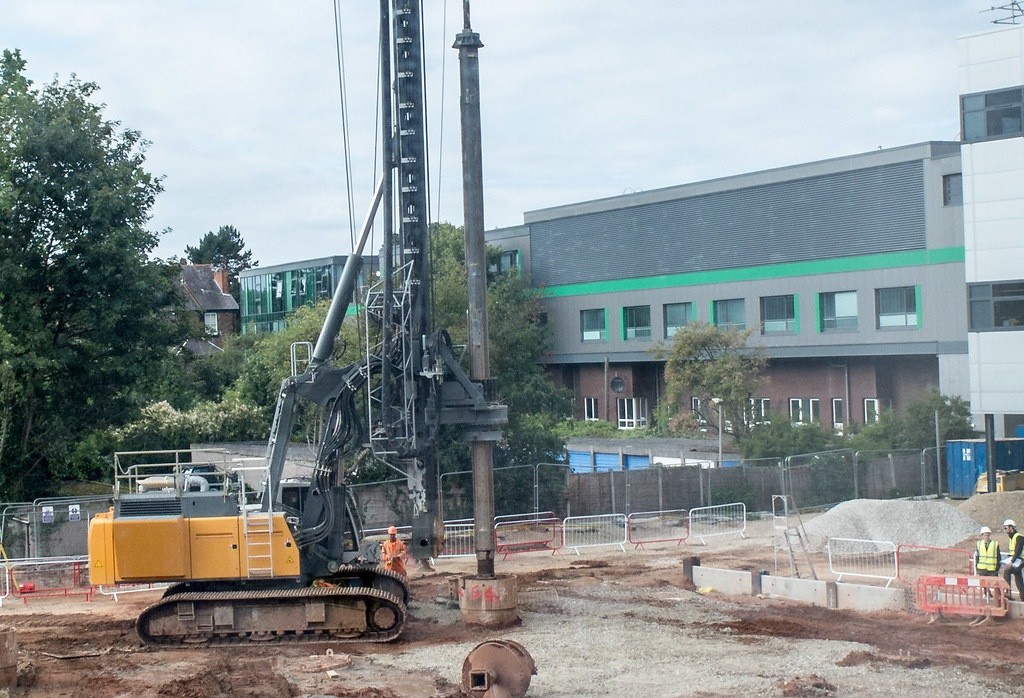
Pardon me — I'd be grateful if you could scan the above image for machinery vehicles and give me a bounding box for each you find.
[83,1,527,649]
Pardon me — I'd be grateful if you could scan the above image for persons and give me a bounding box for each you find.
[975,526,1001,597]
[383,524,407,575]
[1003,519,1024,601]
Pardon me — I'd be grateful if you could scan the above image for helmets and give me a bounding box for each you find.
[388,526,397,534]
[1003,519,1017,527]
[980,526,992,535]
